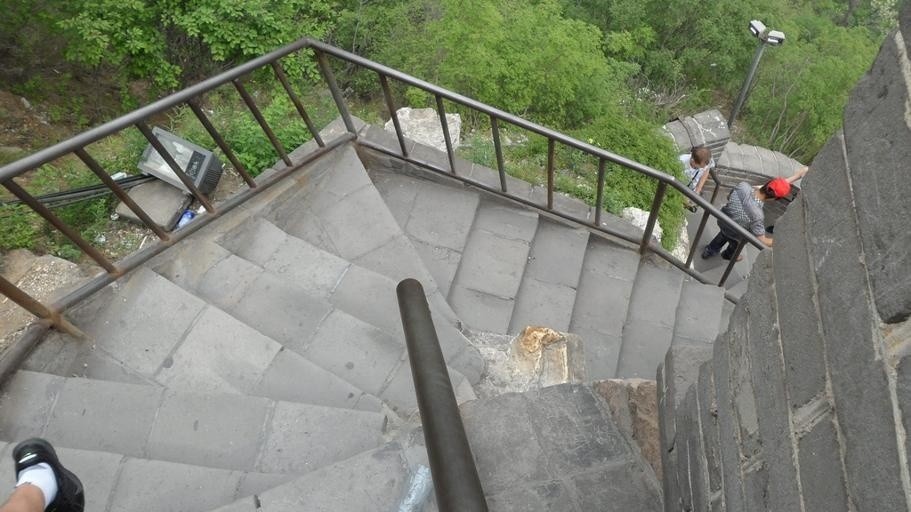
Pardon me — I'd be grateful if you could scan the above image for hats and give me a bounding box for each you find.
[767,178,790,197]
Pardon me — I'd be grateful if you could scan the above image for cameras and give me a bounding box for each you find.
[689,206,698,212]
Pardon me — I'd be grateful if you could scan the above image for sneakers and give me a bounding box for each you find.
[11,438,83,512]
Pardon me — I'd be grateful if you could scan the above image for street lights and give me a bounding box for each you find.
[729,20,787,135]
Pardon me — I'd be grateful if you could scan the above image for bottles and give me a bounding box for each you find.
[176,208,197,230]
[109,171,128,182]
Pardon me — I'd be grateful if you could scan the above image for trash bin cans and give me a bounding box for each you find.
[136,126,223,194]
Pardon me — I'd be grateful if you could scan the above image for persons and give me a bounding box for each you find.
[678,144,713,210]
[2,436,86,510]
[754,166,817,234]
[701,168,795,266]
